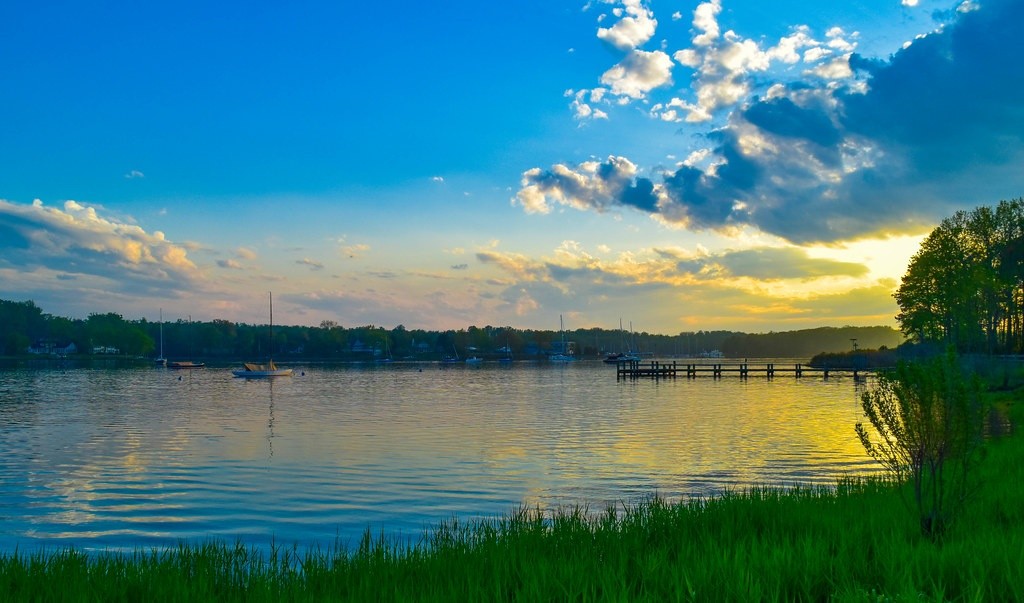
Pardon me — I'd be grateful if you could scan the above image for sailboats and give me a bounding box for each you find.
[153,307,167,365]
[672,337,725,358]
[495,336,512,363]
[547,315,577,362]
[603,319,655,364]
[375,336,395,362]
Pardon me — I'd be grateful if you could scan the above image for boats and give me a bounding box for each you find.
[441,344,461,362]
[169,361,205,367]
[231,358,292,375]
[465,355,484,364]
